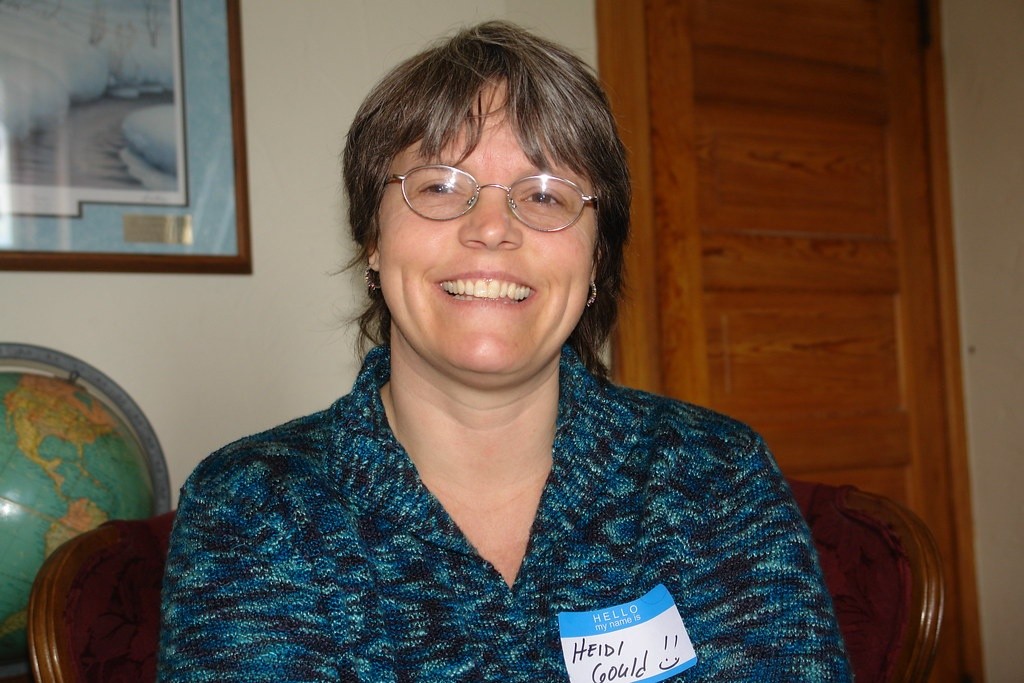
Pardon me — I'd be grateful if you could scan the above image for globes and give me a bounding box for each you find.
[0,338,173,683]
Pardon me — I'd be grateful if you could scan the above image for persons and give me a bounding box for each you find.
[154,18,856,683]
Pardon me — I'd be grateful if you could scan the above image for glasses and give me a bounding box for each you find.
[385,165,600,231]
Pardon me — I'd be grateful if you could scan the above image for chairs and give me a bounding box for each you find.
[786,481,946,683]
[28,509,176,683]
